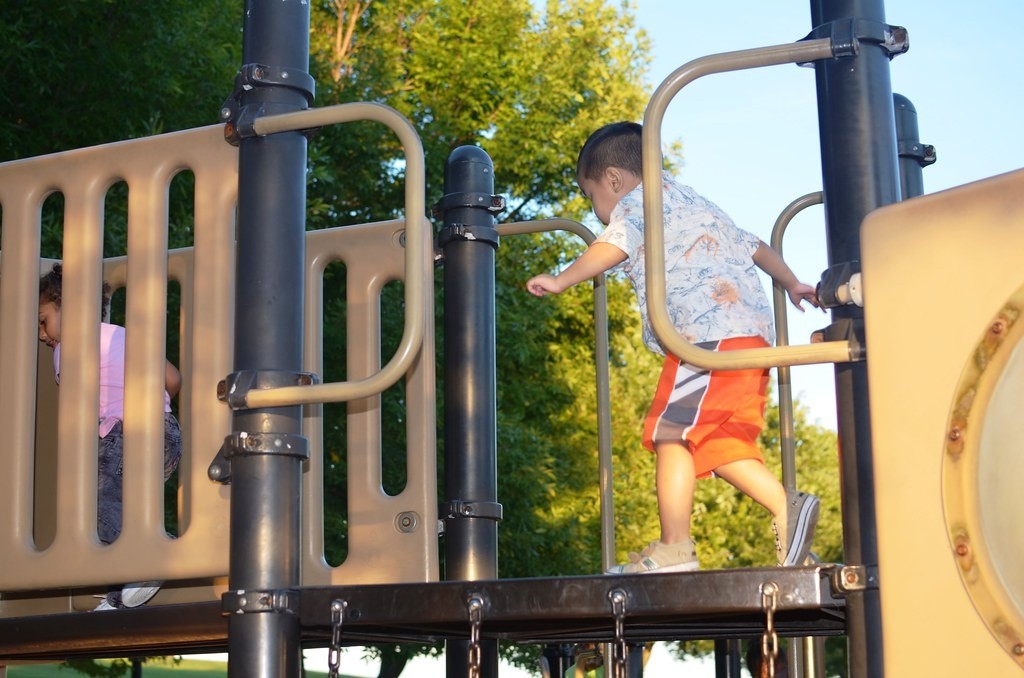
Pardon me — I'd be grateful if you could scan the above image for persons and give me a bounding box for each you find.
[525,119,829,576]
[38,263,184,611]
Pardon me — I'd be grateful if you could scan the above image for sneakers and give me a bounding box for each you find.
[601,541,700,575]
[85,595,118,610]
[774,491,820,568]
[121,531,178,609]
